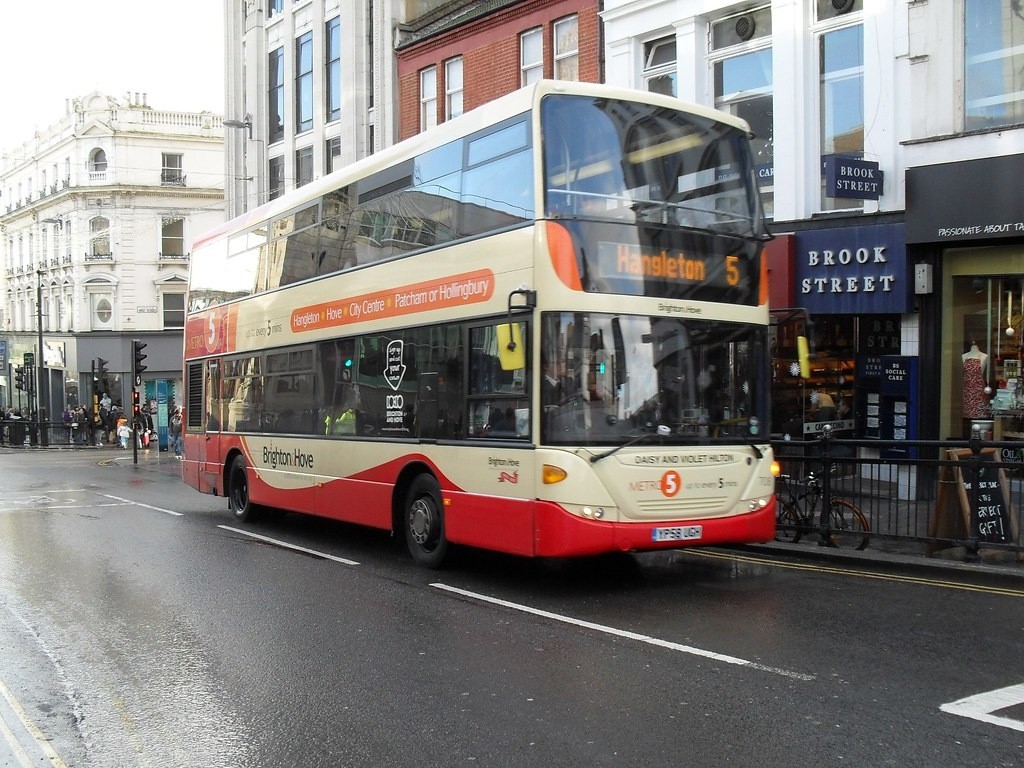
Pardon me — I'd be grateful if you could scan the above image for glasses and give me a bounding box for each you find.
[545,360,565,365]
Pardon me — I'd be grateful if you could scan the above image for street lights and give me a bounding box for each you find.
[31,313,51,447]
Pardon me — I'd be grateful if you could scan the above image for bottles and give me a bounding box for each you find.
[1006,363,1016,378]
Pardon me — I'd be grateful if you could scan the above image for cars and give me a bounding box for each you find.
[182,80,777,569]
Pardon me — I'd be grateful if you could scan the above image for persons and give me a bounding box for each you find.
[837,399,854,447]
[0,405,38,447]
[810,388,836,435]
[62,403,154,449]
[100,393,112,411]
[169,405,182,459]
[962,345,990,418]
[542,350,603,425]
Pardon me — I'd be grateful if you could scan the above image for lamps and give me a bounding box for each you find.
[223,119,252,139]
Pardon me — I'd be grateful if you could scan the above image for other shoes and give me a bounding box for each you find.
[176,455,182,460]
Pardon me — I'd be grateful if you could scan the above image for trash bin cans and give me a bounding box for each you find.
[8,415,26,445]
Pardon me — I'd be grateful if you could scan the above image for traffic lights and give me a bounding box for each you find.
[98,358,109,392]
[132,392,140,404]
[132,406,140,418]
[599,362,606,374]
[131,339,147,386]
[15,367,27,391]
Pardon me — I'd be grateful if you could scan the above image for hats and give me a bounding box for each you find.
[174,409,180,413]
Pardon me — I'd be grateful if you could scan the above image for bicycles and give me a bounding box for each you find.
[775,462,869,551]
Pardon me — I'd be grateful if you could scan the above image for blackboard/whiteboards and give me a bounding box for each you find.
[949,448,1019,543]
